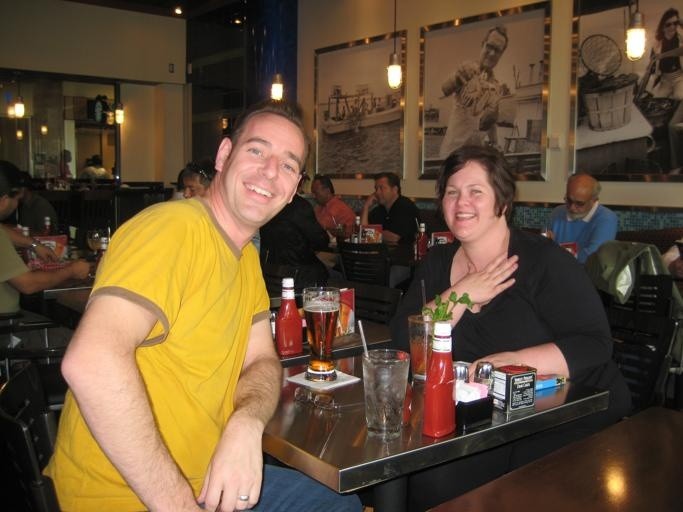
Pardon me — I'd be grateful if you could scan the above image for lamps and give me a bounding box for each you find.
[114,102,125,125]
[106,112,114,126]
[269,72,286,102]
[625,2,647,64]
[6,104,15,120]
[386,1,403,91]
[16,129,23,141]
[15,75,25,118]
[40,124,48,134]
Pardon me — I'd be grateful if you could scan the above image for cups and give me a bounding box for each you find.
[364,349,410,440]
[303,287,341,382]
[87,230,102,256]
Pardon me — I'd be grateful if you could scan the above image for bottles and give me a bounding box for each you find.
[421,321,456,438]
[22,225,32,261]
[416,222,427,257]
[275,277,304,357]
[96,236,109,268]
[354,216,360,234]
[44,215,53,235]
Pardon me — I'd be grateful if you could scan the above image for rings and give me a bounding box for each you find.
[238,495,249,501]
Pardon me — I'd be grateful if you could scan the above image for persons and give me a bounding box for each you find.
[332,92,401,127]
[43,102,365,512]
[387,144,631,435]
[181,159,216,198]
[9,170,59,235]
[79,150,112,181]
[542,173,619,263]
[360,172,423,291]
[258,175,344,297]
[60,150,73,178]
[311,175,355,241]
[441,26,514,159]
[632,8,683,176]
[0,159,91,381]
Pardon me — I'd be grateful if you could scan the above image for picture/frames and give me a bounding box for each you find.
[418,0,553,183]
[567,1,683,183]
[311,29,407,182]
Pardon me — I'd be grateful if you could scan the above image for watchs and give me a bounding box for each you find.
[29,239,39,251]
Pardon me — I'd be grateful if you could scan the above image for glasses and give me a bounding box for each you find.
[484,41,504,56]
[187,162,212,183]
[294,387,364,409]
[564,192,591,208]
[664,20,680,27]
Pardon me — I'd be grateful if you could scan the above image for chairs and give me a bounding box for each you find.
[36,191,72,235]
[336,236,391,287]
[323,277,402,326]
[0,361,67,512]
[78,189,115,249]
[0,321,71,430]
[605,306,680,415]
[600,274,683,411]
